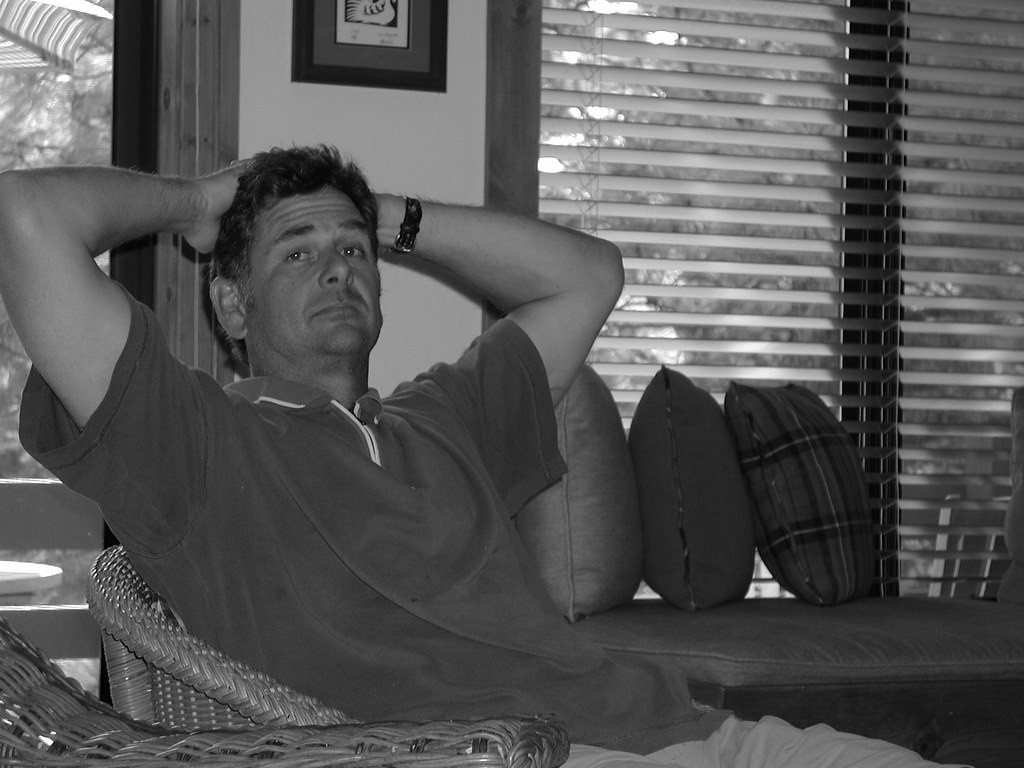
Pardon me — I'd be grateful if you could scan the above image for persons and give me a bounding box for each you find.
[0,146,973,768]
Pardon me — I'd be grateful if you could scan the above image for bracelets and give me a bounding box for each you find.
[386,197,423,254]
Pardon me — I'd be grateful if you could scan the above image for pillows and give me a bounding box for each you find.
[515,363,642,622]
[628,365,755,610]
[723,381,881,612]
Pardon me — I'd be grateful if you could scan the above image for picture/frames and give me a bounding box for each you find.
[290,0,447,94]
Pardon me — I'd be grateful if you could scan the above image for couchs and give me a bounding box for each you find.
[567,388,1024,768]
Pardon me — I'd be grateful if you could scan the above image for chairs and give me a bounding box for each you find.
[85,547,367,735]
